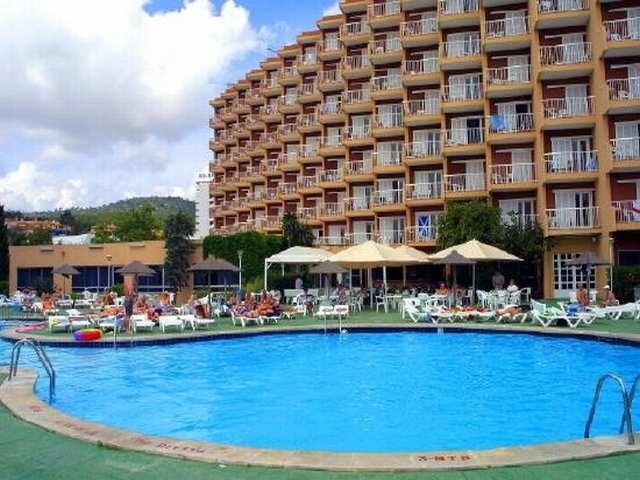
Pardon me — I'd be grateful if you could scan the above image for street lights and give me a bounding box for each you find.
[105,254,114,291]
[607,235,614,293]
[237,249,246,291]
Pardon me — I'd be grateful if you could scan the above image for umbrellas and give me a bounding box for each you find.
[185,255,244,290]
[309,238,525,305]
[50,264,81,297]
[114,260,157,294]
[564,251,612,300]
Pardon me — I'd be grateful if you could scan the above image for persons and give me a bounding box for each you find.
[492,270,504,290]
[495,305,530,317]
[575,282,616,308]
[2,283,315,333]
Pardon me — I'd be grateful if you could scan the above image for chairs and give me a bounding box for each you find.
[0,288,640,334]
